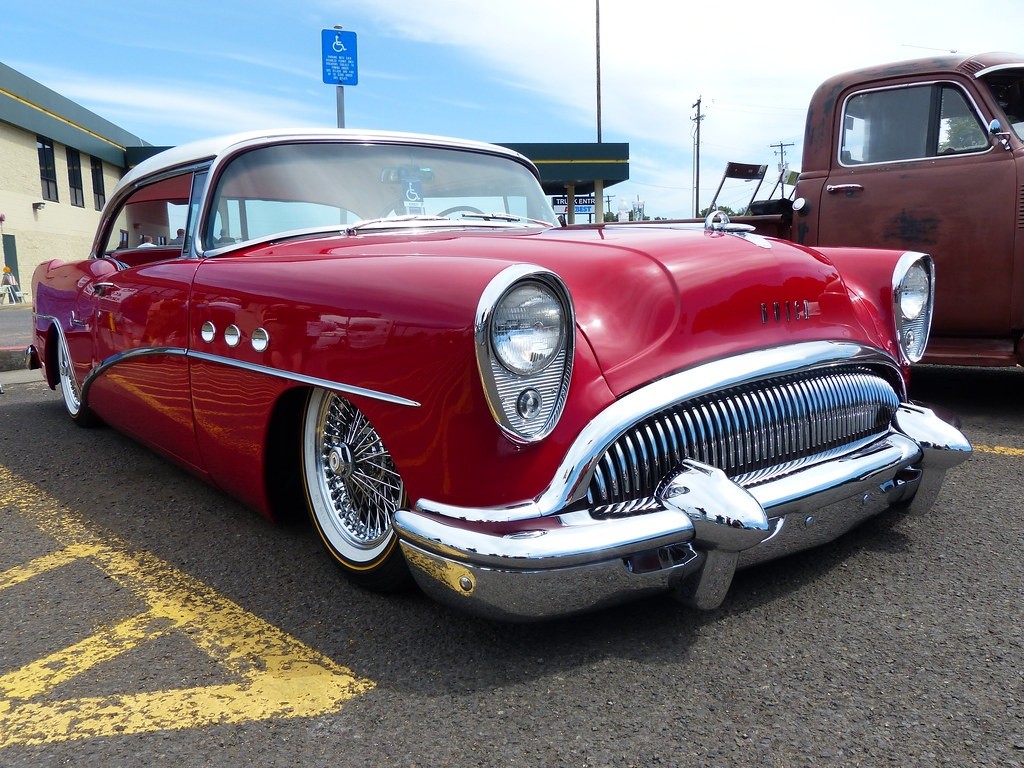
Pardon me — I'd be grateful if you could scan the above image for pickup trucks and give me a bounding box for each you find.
[595,50,1024,372]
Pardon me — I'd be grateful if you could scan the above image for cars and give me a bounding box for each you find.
[26,127,974,628]
